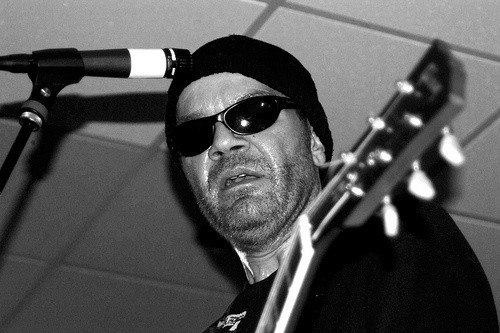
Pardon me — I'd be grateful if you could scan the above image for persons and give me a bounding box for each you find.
[166,36,500,333]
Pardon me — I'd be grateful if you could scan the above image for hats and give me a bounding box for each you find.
[165,35,333,168]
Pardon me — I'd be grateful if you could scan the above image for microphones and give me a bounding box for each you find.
[0,48,192,79]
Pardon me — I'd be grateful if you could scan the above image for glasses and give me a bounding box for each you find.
[169,95,306,157]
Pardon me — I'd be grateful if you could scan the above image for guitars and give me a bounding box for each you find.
[255,39,466,333]
